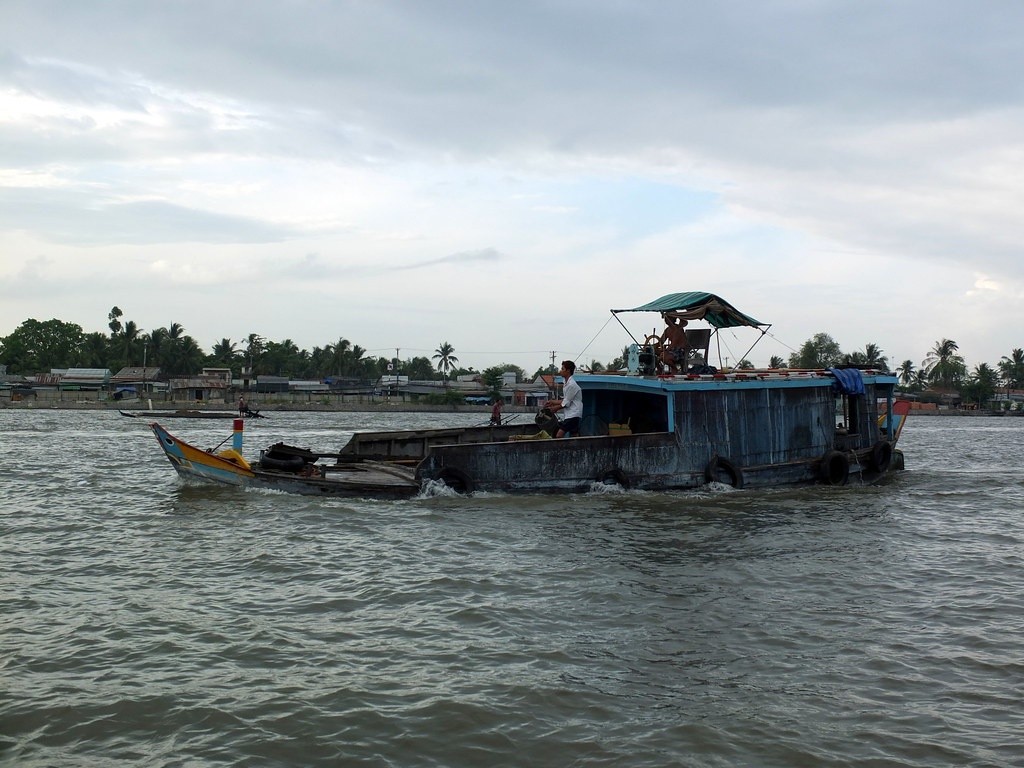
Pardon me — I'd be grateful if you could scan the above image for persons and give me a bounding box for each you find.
[643,310,687,375]
[546,360,583,438]
[239,398,244,416]
[489,399,502,426]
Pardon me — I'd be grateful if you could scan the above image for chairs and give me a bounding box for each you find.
[239,409,251,417]
[664,327,713,374]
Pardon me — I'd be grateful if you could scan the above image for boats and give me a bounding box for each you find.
[151,290,912,493]
[119,410,260,418]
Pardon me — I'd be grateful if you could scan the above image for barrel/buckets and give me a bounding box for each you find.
[533,408,560,435]
